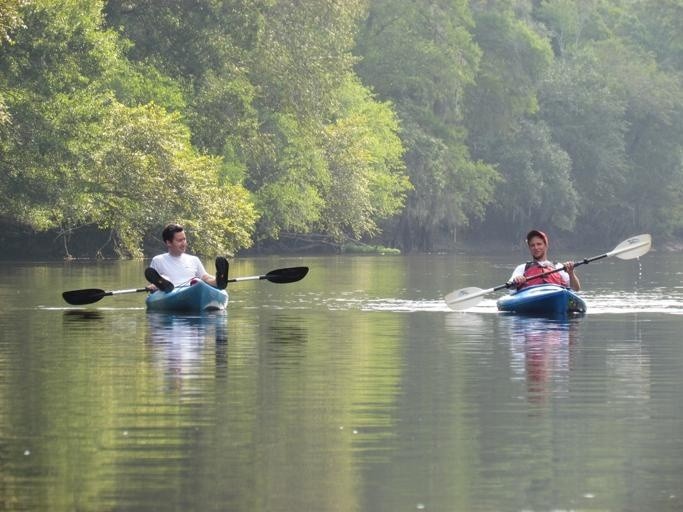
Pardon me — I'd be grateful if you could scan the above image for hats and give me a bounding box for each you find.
[526,230,549,250]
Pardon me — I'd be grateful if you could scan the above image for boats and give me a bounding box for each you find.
[143,310,229,375]
[506,308,577,380]
[495,283,587,314]
[144,279,229,315]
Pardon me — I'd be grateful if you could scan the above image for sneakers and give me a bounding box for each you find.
[144,268,174,294]
[215,256,229,290]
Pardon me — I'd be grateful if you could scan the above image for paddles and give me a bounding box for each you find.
[445,234,652,311]
[62,267,308,306]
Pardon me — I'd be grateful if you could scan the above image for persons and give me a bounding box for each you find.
[145,224,228,294]
[507,230,580,294]
